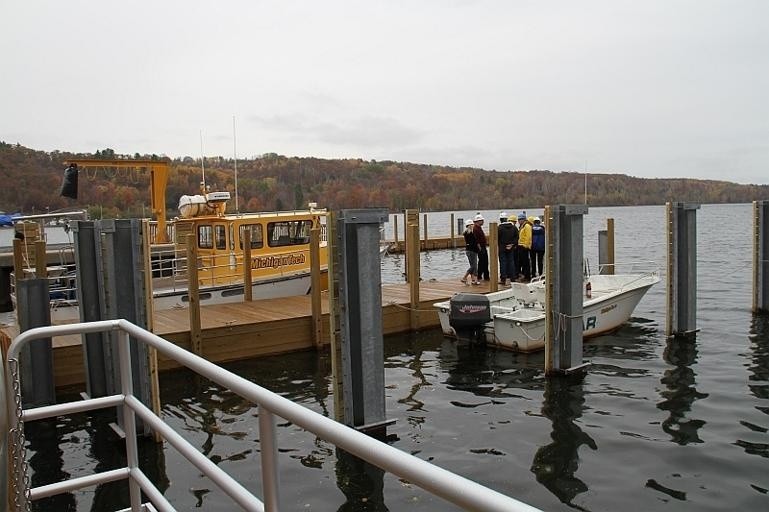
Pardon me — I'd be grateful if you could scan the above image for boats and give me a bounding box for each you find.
[433,261,662,353]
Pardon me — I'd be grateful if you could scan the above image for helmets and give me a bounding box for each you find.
[473,215,484,221]
[466,219,474,227]
[499,213,541,225]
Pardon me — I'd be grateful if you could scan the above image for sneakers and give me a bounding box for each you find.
[461,278,480,286]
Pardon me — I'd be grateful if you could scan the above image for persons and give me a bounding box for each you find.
[461,220,480,286]
[473,215,490,281]
[497,212,545,285]
